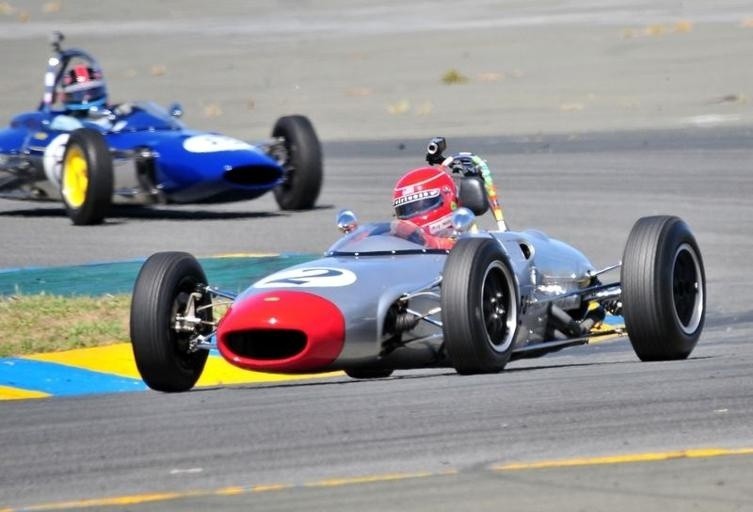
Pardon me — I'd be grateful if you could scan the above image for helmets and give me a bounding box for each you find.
[55,64,108,111]
[392,167,458,235]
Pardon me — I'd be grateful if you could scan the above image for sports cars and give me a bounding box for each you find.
[0,28,326,232]
[124,126,712,396]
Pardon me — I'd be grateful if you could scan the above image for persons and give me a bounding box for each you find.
[333,163,481,251]
[52,62,134,133]
